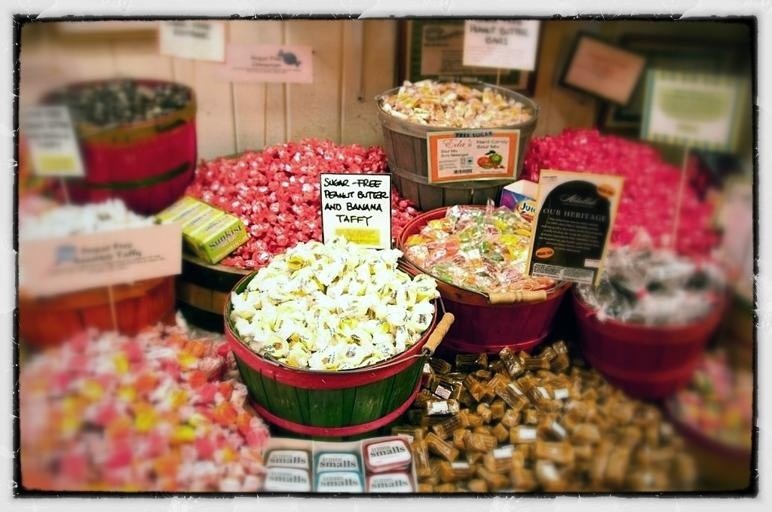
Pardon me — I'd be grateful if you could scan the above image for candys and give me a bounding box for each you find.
[18,77,753,493]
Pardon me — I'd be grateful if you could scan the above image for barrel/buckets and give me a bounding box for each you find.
[714,289,753,377]
[36,78,197,219]
[662,400,750,491]
[173,148,255,334]
[376,80,540,215]
[571,246,734,402]
[393,204,573,363]
[220,266,455,446]
[15,273,177,350]
